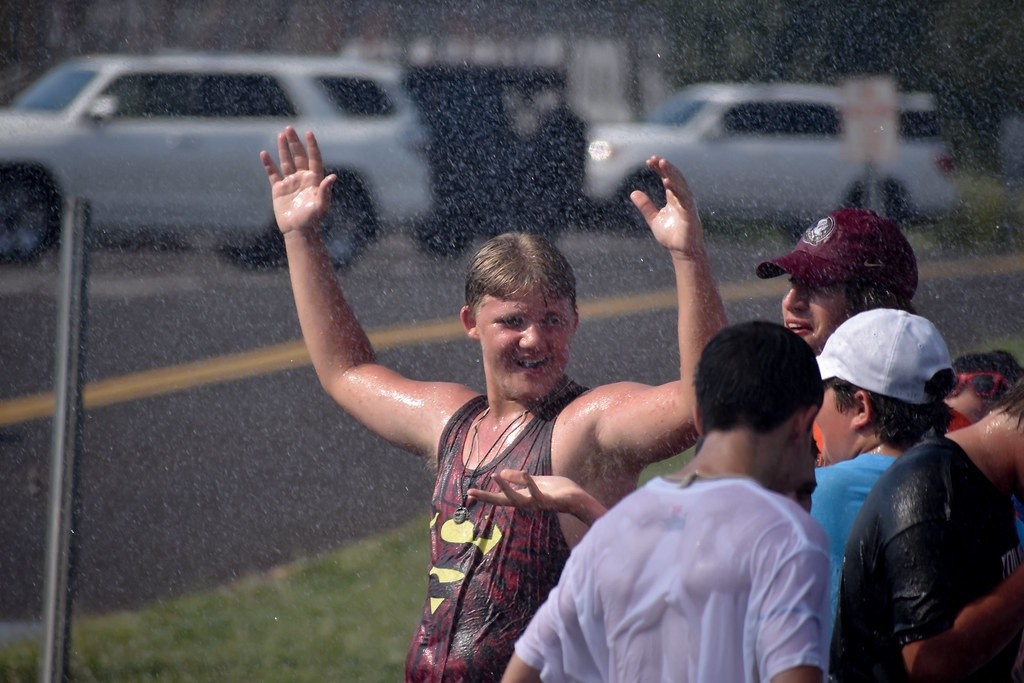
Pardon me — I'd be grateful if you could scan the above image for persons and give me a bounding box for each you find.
[467,208,918,527]
[943,350,1024,433]
[810,309,1024,643]
[260,125,729,683]
[502,322,831,683]
[826,371,1024,683]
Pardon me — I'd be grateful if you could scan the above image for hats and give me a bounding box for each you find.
[815,307,956,404]
[755,207,919,302]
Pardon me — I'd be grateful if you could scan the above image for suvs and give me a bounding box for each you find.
[0,52,441,265]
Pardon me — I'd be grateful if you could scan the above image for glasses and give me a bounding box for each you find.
[946,370,1010,399]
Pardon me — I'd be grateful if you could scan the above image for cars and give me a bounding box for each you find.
[578,77,964,235]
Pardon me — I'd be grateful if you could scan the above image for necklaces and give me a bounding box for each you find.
[454,376,574,524]
